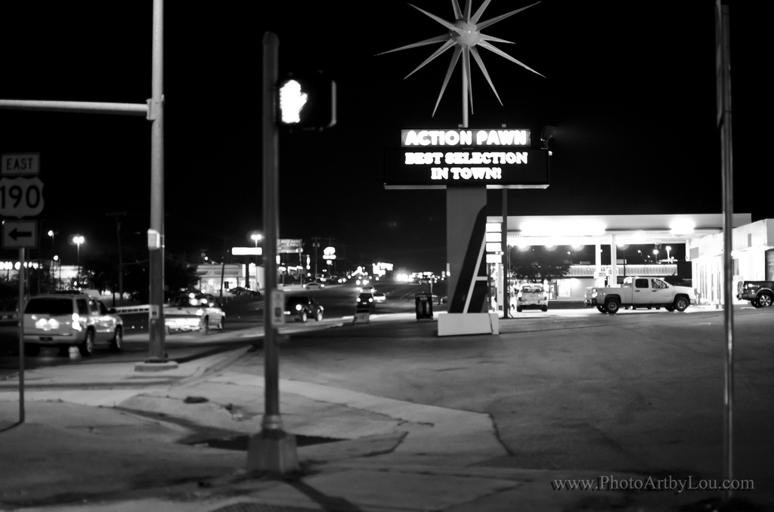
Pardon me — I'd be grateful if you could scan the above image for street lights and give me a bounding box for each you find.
[665,245,672,264]
[539,132,552,198]
[653,249,659,263]
[72,234,84,290]
[250,233,263,265]
[48,228,56,286]
[54,254,61,291]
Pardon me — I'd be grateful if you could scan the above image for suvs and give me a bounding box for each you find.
[17,290,126,356]
[517,283,549,312]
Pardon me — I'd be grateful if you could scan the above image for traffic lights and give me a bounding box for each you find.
[269,76,338,130]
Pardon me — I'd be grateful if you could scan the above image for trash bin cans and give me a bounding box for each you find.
[415,293,433,322]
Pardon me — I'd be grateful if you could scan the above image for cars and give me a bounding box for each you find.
[163,288,228,335]
[336,263,434,312]
[283,295,324,324]
[300,281,326,291]
[229,287,260,297]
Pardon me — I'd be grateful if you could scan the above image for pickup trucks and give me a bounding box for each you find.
[737,278,773,308]
[584,276,696,315]
